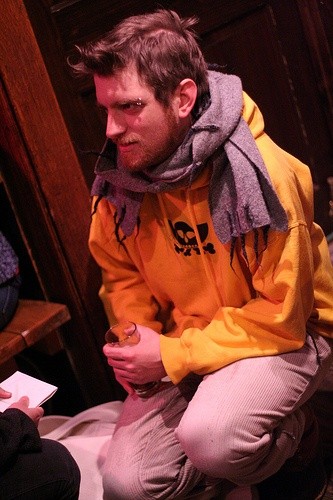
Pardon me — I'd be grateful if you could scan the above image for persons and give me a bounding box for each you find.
[0,384,126,500]
[65,9,333,500]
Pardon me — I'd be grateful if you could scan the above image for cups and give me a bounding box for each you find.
[105,321,160,397]
